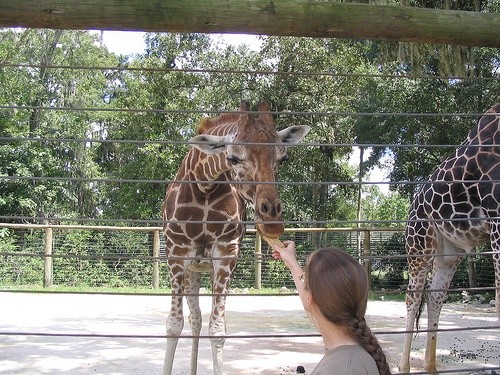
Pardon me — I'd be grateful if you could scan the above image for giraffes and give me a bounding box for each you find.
[160,100,314,374]
[397,100,500,374]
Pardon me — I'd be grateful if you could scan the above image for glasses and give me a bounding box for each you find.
[298,272,305,284]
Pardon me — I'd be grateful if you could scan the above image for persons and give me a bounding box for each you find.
[272,241,392,375]
